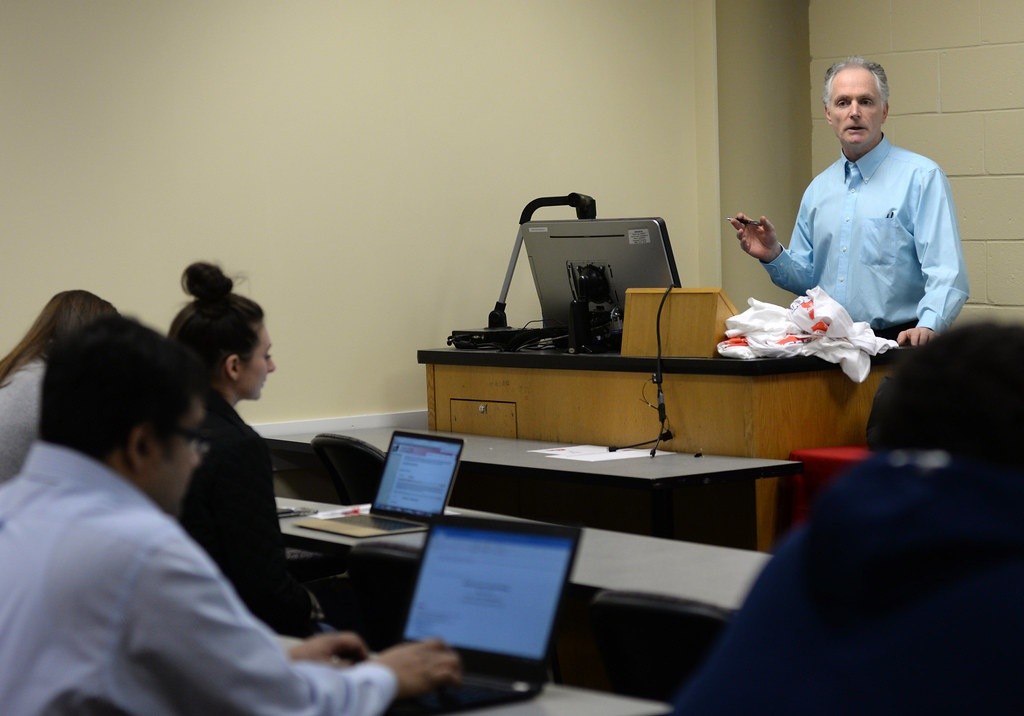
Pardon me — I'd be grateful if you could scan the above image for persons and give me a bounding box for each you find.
[156,258,394,650]
[728,55,970,347]
[0,317,466,716]
[668,318,1023,716]
[0,288,125,497]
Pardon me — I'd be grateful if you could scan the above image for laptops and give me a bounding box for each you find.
[294,430,465,538]
[374,513,582,716]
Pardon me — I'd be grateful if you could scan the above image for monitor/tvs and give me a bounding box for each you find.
[521,217,682,354]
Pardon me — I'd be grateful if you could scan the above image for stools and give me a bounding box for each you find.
[787,444,877,541]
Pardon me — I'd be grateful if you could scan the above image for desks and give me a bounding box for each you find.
[271,492,774,703]
[258,630,673,715]
[258,424,803,556]
[417,338,926,558]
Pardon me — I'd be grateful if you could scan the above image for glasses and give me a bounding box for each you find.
[152,419,209,445]
